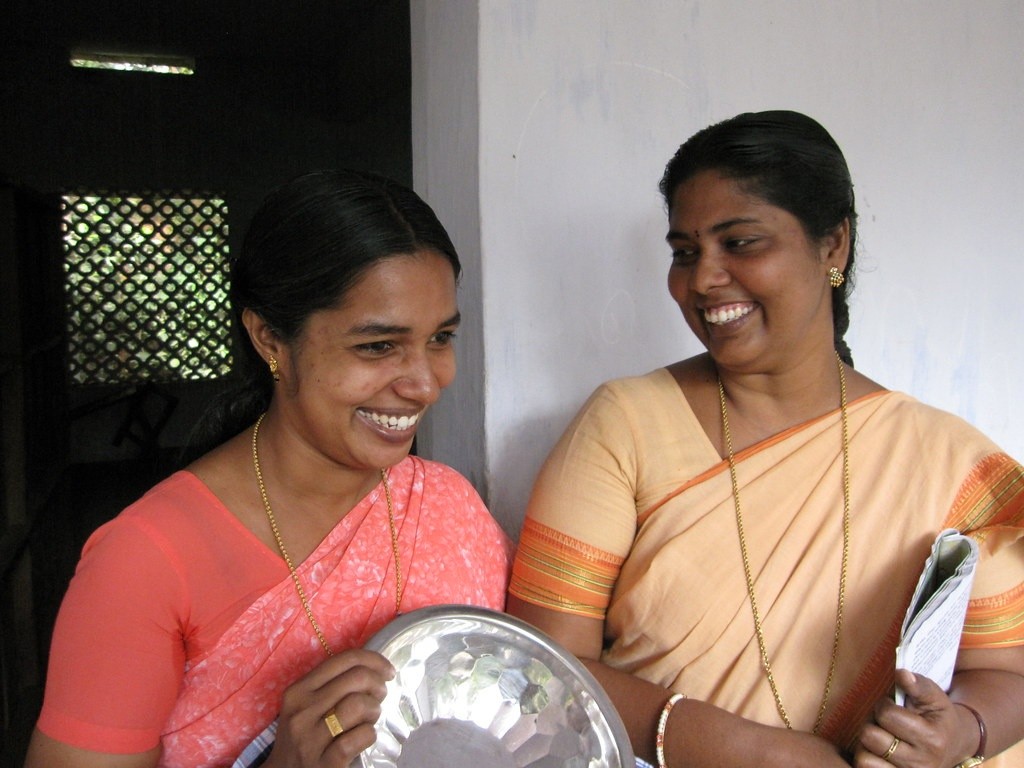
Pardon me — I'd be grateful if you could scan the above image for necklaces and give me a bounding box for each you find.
[252,413,401,657]
[717,352,849,735]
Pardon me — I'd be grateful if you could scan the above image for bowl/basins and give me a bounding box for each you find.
[346,604,637,768]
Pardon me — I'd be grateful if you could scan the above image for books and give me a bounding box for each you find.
[894,526,978,705]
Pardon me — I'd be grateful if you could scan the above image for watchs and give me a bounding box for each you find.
[952,702,986,768]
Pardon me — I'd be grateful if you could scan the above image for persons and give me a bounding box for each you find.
[23,172,518,768]
[506,110,1024,768]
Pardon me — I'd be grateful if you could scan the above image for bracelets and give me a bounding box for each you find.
[656,694,687,768]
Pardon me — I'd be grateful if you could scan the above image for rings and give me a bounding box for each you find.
[882,738,898,760]
[323,709,344,739]
